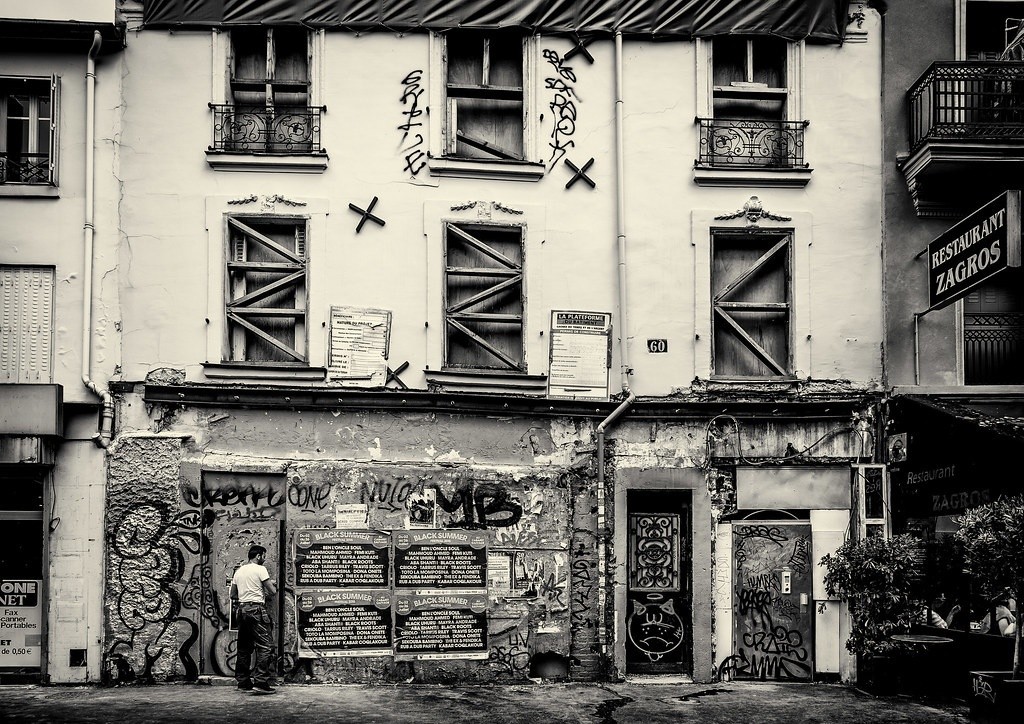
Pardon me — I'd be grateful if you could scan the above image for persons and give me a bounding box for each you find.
[230,545,277,693]
[922,605,961,629]
[981,594,1016,635]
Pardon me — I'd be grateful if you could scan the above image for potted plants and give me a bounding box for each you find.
[947,492,1024,724]
[815,528,928,698]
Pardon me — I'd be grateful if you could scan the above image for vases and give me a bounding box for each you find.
[889,634,953,697]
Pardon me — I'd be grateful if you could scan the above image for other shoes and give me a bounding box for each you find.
[252,681,276,694]
[238,681,253,692]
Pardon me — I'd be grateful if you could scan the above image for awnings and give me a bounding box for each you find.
[897,397,1024,519]
